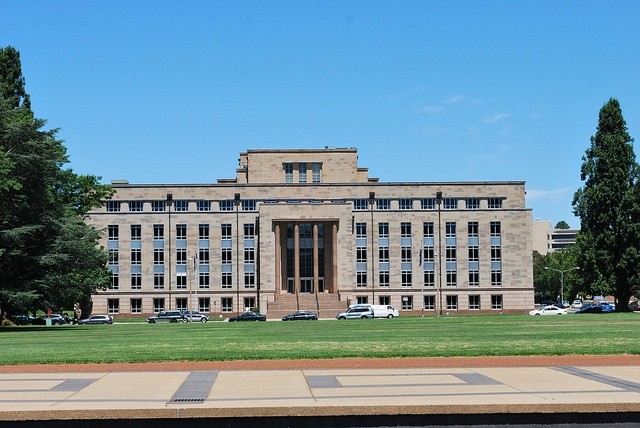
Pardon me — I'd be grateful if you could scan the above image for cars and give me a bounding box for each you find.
[183,310,210,323]
[282,310,318,321]
[11,313,75,326]
[229,311,266,322]
[529,306,568,316]
[79,314,112,325]
[563,300,618,312]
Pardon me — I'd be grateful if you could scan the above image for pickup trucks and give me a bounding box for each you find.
[628,301,640,310]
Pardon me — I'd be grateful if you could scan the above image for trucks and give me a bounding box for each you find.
[372,305,400,318]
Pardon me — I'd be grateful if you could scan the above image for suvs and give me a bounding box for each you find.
[147,310,184,323]
[336,308,373,320]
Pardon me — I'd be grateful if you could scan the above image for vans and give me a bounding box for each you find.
[348,304,372,308]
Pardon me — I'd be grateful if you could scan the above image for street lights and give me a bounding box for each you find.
[545,266,580,304]
